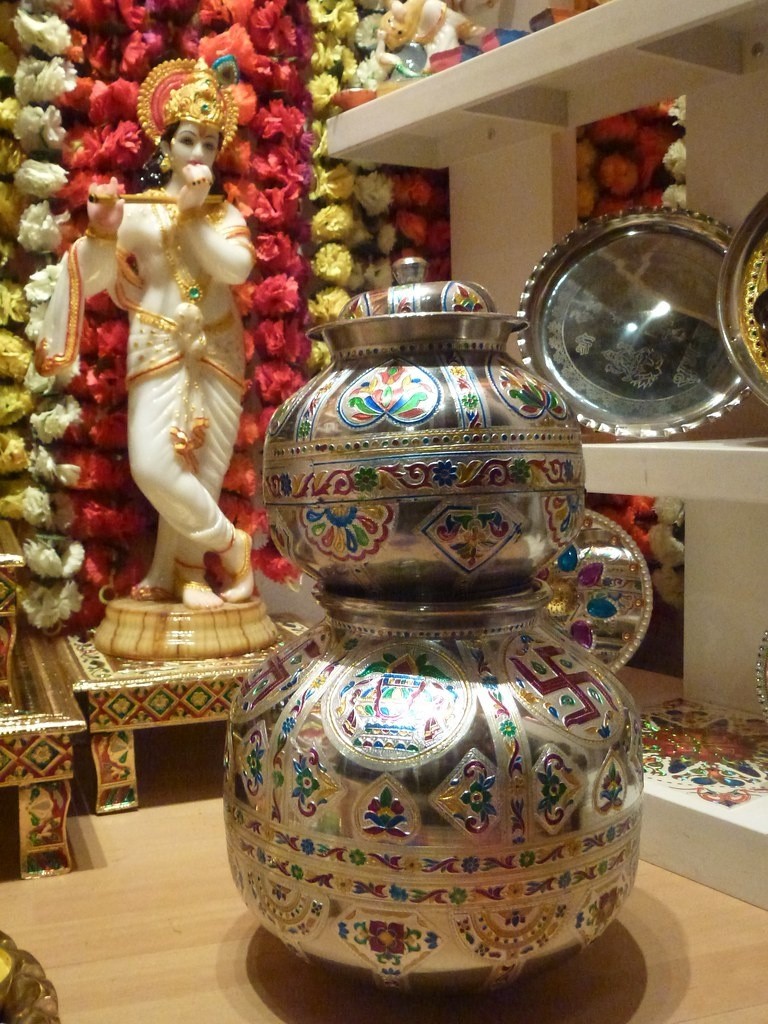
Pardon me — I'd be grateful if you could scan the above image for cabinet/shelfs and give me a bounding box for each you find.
[322,0,768,914]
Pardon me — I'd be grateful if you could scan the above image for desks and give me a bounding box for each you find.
[0,787,768,1024]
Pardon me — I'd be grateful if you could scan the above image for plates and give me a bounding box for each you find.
[515,191,768,442]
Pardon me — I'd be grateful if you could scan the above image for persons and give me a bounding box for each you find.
[34,58,257,608]
